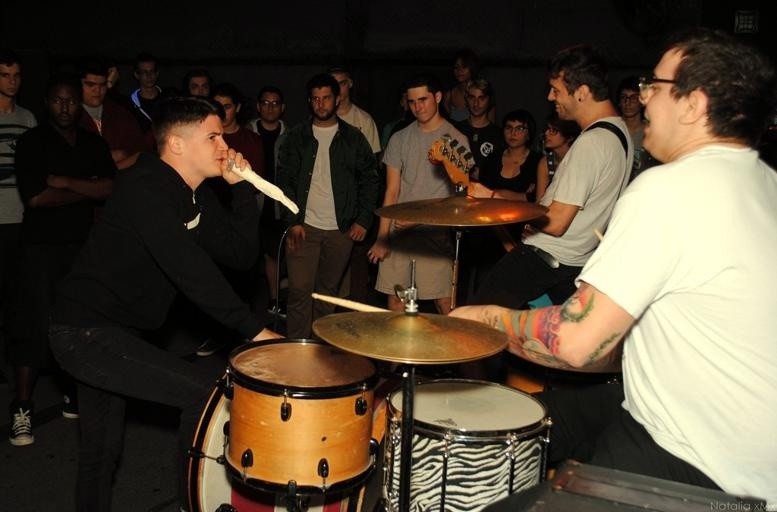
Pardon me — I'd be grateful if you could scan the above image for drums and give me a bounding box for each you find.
[185,374,388,512]
[225,337,379,511]
[380,378,552,512]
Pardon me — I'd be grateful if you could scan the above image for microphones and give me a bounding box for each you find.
[221,154,300,218]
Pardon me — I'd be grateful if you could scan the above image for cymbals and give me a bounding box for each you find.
[312,308,507,365]
[372,191,550,226]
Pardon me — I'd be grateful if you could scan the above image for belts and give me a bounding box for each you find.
[530,246,560,269]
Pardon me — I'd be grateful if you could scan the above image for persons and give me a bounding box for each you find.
[50,96,286,512]
[448,27,777,511]
[0,36,661,445]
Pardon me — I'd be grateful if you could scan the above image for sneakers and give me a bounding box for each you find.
[62,395,78,418]
[196,342,223,358]
[9,400,34,446]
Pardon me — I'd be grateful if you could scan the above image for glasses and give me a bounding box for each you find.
[544,127,560,135]
[639,76,677,100]
[262,100,279,107]
[505,125,528,133]
